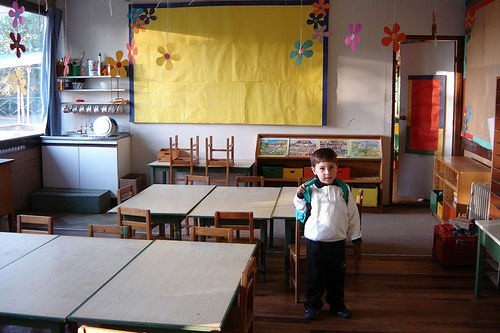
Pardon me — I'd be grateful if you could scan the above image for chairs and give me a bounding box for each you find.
[117,134,363,307]
[16,214,53,235]
[78,325,137,333]
[190,226,232,243]
[88,224,131,239]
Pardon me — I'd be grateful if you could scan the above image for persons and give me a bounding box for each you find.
[293,147,362,322]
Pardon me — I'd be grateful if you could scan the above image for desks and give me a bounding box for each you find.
[475,219,500,300]
[148,160,255,187]
[0,184,300,333]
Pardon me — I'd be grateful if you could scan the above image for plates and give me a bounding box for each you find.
[93,115,118,137]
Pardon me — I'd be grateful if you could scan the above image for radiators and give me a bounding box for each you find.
[469,181,491,221]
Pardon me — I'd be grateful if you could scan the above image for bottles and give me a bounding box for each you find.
[56,52,110,90]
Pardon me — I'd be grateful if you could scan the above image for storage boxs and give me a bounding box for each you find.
[32,188,111,214]
[433,223,477,265]
[262,164,378,207]
[120,173,146,194]
[430,189,443,218]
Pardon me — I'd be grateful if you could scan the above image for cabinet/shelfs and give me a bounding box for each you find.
[39,133,131,198]
[255,134,384,213]
[0,159,18,232]
[56,75,127,105]
[430,156,491,224]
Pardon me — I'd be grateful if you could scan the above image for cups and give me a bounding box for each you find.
[63,104,123,114]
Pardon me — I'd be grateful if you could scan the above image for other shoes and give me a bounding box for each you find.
[330,304,351,319]
[304,309,318,319]
